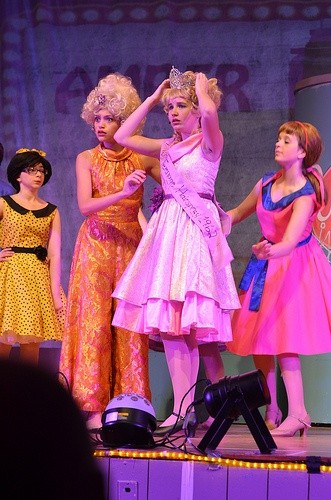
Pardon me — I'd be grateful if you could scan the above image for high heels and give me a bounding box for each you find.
[268,414,311,438]
[152,411,198,438]
[264,408,283,430]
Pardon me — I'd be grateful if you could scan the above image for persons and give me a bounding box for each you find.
[59,72,152,432]
[110,66,241,438]
[0,149,67,366]
[0,354,109,500]
[224,120,331,437]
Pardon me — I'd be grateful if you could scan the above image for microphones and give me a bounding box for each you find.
[169,122,171,124]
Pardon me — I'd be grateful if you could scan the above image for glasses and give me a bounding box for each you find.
[23,167,48,176]
